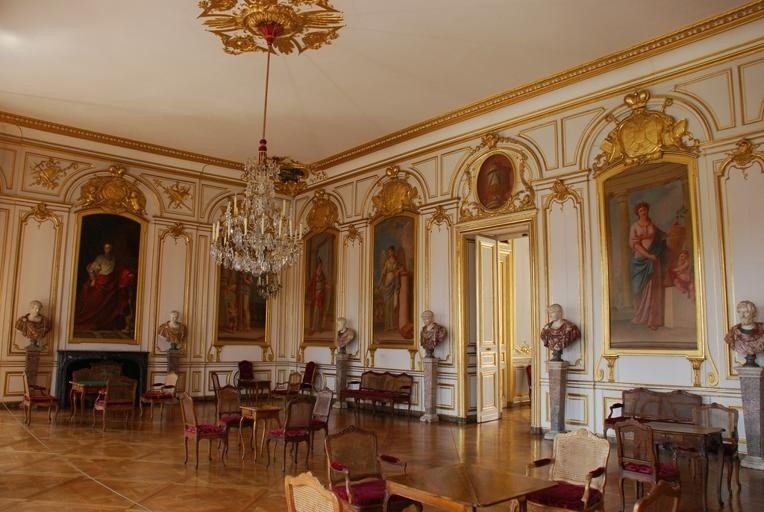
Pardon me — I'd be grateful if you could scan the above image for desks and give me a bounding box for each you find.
[381,462,557,512]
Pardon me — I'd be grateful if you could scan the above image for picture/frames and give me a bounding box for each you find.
[367,208,419,350]
[590,152,708,359]
[210,250,274,347]
[65,206,149,343]
[297,224,340,348]
[469,151,517,212]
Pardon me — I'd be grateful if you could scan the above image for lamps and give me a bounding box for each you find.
[195,0,342,279]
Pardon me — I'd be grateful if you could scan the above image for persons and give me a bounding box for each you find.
[13,299,53,342]
[539,303,579,353]
[335,316,356,348]
[418,311,446,350]
[86,240,120,311]
[156,310,184,349]
[723,300,764,355]
[628,202,664,331]
[373,246,401,328]
[305,256,327,333]
[669,250,692,296]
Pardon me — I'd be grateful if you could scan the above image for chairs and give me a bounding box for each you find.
[177,358,412,473]
[602,386,745,511]
[321,425,420,511]
[510,428,610,511]
[282,473,343,511]
[21,364,178,433]
